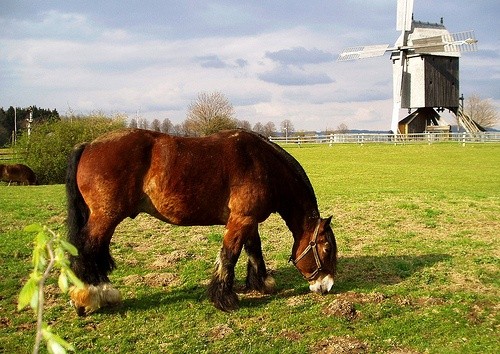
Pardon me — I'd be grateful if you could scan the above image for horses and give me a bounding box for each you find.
[0,163,37,187]
[66,129,337,313]
